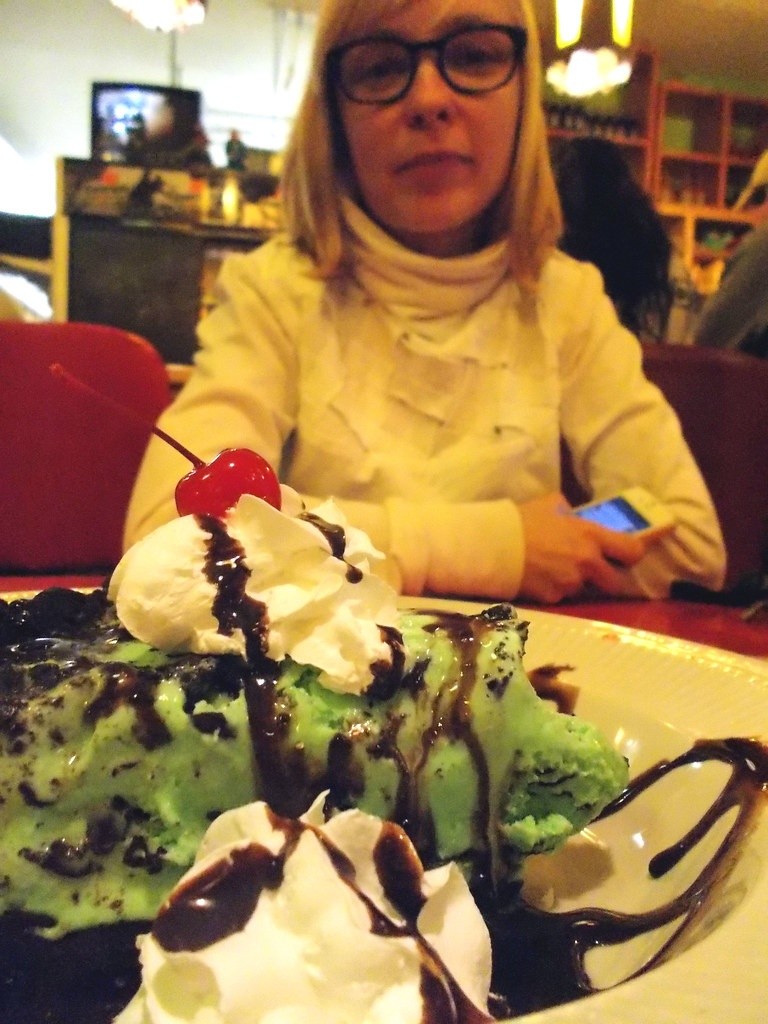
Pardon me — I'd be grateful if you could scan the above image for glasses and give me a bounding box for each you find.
[326,22,526,104]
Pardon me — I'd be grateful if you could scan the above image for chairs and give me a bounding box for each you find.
[0,316,175,581]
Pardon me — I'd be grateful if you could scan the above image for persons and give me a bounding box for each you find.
[691,149,768,361]
[122,0,729,606]
[182,132,211,169]
[547,135,673,341]
[122,113,155,165]
[226,129,248,172]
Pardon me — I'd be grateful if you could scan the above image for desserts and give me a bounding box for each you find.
[0,358,766,1024]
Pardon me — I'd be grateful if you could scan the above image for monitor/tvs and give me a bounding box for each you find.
[90,81,201,163]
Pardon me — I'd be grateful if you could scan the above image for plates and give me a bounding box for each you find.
[1,585,768,1024]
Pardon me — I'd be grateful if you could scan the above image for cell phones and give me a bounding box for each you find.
[573,486,674,544]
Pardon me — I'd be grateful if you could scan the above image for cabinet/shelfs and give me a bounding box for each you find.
[539,41,658,197]
[652,76,768,353]
